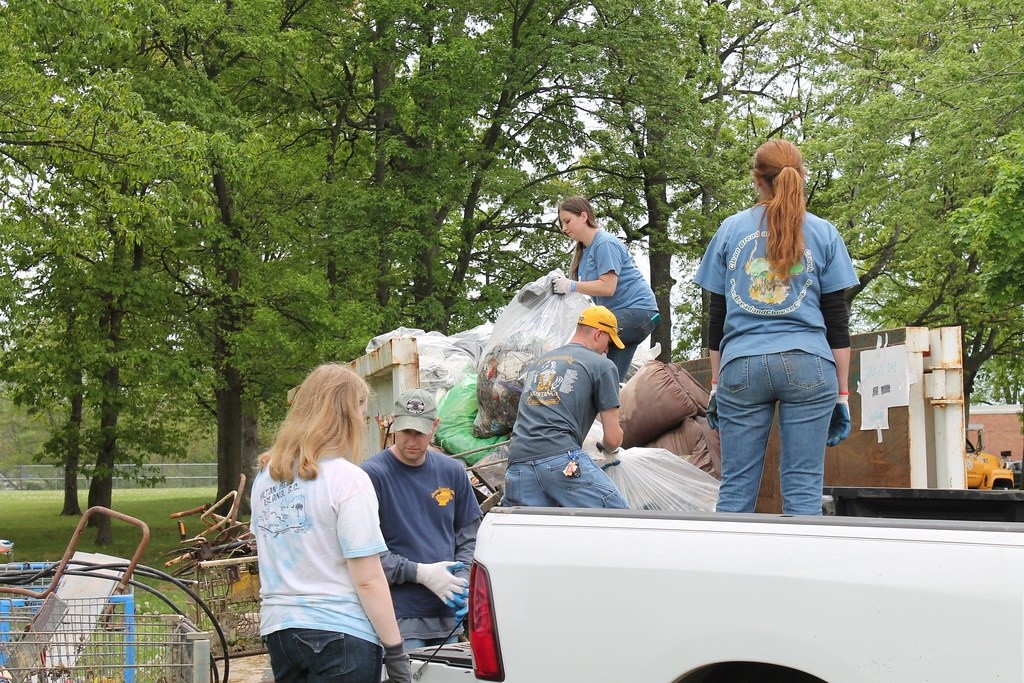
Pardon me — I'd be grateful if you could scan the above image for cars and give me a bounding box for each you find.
[1010,460,1024,490]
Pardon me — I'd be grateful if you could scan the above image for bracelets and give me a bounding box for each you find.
[571,281,576,292]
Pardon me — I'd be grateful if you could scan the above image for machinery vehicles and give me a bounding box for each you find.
[965,425,1015,490]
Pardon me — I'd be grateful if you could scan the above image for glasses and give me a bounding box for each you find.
[599,322,624,337]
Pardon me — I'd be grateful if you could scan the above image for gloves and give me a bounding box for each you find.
[707,381,722,431]
[381,637,413,683]
[417,561,469,629]
[553,277,576,295]
[826,391,851,449]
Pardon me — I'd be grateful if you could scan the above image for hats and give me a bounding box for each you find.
[394,388,438,435]
[577,305,625,350]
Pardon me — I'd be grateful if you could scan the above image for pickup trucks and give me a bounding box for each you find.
[389,487,1024,682]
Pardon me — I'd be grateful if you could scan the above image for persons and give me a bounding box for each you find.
[503,306,633,510]
[694,139,860,516]
[358,388,484,650]
[552,197,662,389]
[250,365,413,683]
